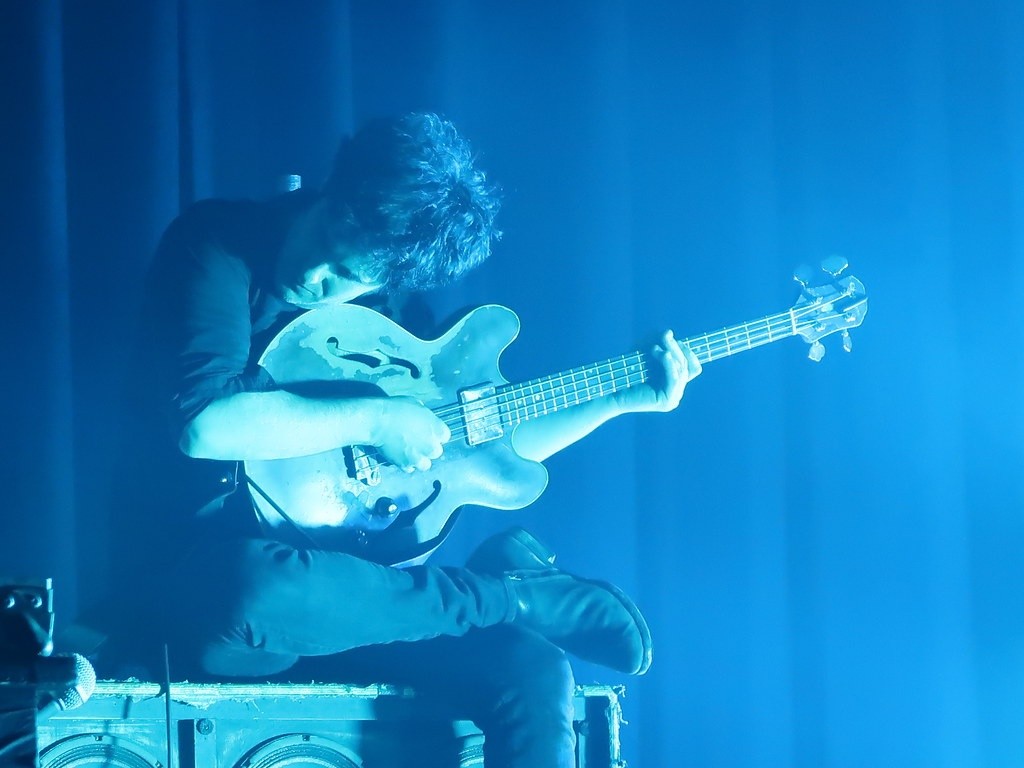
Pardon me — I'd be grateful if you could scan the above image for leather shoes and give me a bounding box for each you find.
[467,528,652,676]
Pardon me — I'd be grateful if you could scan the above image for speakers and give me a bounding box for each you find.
[38,685,625,768]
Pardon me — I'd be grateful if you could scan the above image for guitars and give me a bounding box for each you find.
[241,275,869,565]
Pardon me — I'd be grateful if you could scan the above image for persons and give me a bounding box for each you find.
[112,111,703,768]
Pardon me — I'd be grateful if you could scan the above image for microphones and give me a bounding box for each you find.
[39,652,96,724]
